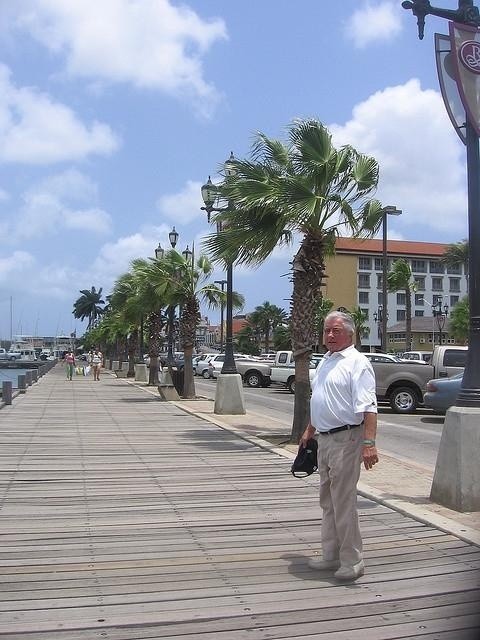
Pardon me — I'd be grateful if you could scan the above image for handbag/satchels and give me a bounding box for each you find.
[75,366,92,377]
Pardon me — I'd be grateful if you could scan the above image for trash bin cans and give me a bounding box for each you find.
[171,370,184,396]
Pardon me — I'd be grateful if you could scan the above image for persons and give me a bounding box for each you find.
[92,347,103,382]
[299,308,380,583]
[61,348,76,381]
[86,350,93,367]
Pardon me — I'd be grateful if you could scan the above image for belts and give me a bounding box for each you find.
[319,420,364,434]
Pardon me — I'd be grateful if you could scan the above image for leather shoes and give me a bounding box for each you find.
[335,559,364,579]
[309,560,339,570]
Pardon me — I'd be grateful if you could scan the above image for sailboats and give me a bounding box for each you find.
[7,296,76,363]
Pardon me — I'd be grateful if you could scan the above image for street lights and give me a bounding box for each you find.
[373,303,390,346]
[418,298,435,352]
[380,205,403,355]
[431,296,449,344]
[199,149,241,374]
[154,226,192,367]
[214,280,229,353]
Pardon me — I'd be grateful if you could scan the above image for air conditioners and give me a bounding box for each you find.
[420,338,425,344]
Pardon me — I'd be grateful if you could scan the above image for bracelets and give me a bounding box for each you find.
[361,439,376,448]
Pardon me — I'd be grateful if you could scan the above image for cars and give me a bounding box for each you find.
[422,372,466,412]
[261,350,434,364]
[141,349,278,378]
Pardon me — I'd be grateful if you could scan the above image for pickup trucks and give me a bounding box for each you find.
[0,347,22,362]
[270,357,325,394]
[368,345,470,412]
[234,350,294,388]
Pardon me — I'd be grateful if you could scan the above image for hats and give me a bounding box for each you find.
[291,438,318,478]
